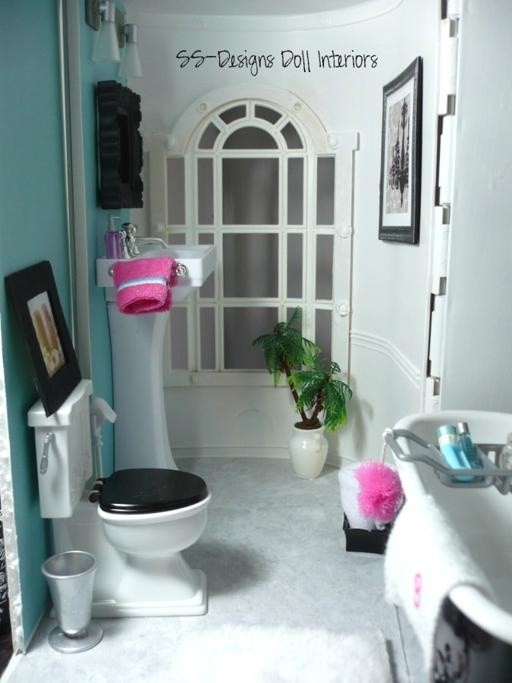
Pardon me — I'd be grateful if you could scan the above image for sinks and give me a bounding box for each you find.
[98,244,215,305]
[391,409,512,655]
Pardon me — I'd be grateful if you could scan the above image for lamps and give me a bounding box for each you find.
[85,0,145,80]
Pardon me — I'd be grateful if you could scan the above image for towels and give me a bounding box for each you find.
[113,256,177,314]
[380,493,497,682]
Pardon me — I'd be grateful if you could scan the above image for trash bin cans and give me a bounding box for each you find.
[41,549,104,656]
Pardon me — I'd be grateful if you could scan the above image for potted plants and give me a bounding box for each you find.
[251,305,355,477]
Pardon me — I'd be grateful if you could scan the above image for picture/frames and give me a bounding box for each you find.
[3,258,84,418]
[377,55,423,245]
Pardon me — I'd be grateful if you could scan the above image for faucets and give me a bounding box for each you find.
[106,214,168,249]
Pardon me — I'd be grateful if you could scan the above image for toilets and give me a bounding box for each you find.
[28,379,212,617]
